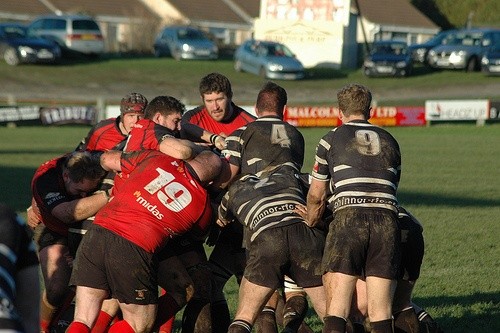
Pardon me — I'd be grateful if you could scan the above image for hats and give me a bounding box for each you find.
[117,92,148,120]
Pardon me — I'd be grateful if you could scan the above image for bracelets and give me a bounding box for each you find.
[102,189,111,203]
[26,205,33,211]
[210,133,219,145]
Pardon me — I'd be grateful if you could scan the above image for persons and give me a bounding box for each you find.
[218,162,353,333]
[349,204,425,333]
[0,72,313,333]
[294,83,402,333]
[281,172,439,333]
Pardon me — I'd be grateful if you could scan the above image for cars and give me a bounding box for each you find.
[481,34,500,73]
[153,25,219,61]
[426,27,500,72]
[407,29,456,64]
[0,23,63,67]
[363,39,413,78]
[234,39,306,82]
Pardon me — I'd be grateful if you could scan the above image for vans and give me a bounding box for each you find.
[26,14,106,59]
